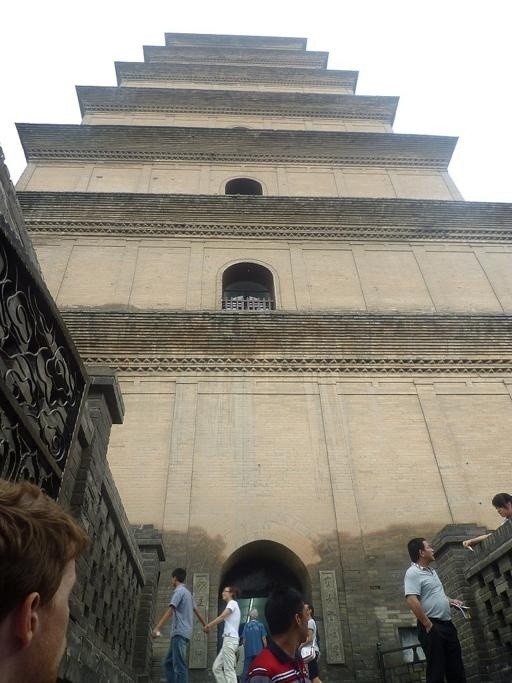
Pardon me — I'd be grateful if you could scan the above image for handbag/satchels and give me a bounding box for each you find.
[300,645,316,663]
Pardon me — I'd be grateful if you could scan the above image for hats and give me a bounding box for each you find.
[248,609,258,619]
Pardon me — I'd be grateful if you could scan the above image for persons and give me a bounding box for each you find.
[1,477,92,683]
[404,537,467,683]
[240,608,268,683]
[247,586,309,683]
[203,586,241,683]
[151,567,206,683]
[298,602,322,683]
[235,644,245,683]
[462,493,512,549]
